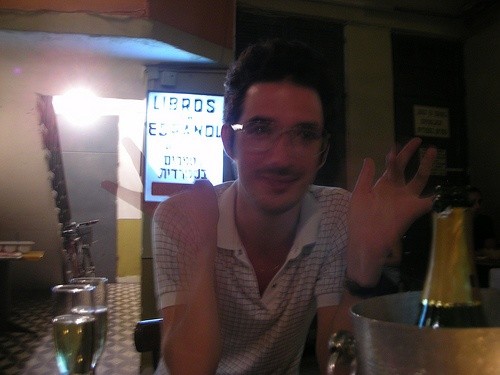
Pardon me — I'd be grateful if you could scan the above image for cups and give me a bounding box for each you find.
[52,277,108,375]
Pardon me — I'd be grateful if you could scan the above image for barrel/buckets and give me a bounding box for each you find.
[325,287,500,375]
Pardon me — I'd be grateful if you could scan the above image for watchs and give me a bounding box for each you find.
[343,267,391,300]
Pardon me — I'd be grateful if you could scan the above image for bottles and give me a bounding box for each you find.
[417,184,488,328]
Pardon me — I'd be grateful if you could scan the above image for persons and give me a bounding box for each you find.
[100,39,440,375]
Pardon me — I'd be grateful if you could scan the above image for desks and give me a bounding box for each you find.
[0,251,45,333]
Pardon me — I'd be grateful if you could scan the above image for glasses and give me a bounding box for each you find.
[231,119,329,158]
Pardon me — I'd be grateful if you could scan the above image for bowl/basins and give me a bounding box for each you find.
[0,240,36,253]
